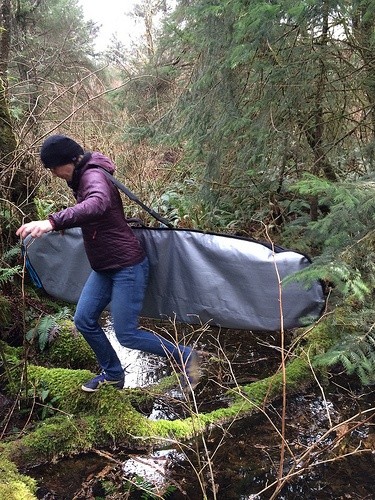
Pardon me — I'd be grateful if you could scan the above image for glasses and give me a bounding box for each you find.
[46,167,56,171]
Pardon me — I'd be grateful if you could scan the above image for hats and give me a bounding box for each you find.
[40,134,84,168]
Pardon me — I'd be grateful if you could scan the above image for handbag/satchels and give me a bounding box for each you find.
[21,166,326,332]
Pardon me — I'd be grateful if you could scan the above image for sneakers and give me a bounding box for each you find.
[180,349,202,393]
[81,371,124,392]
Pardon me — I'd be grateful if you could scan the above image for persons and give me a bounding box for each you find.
[16,135,201,392]
